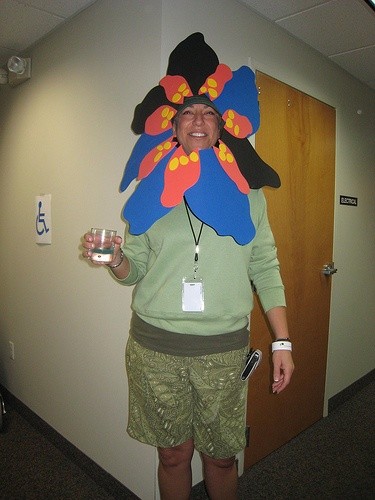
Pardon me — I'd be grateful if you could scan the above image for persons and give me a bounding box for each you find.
[82,32,294,500]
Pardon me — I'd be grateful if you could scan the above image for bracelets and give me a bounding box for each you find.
[271,338,293,352]
[108,248,124,268]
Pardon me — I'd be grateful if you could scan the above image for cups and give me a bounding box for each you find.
[86,228,115,266]
